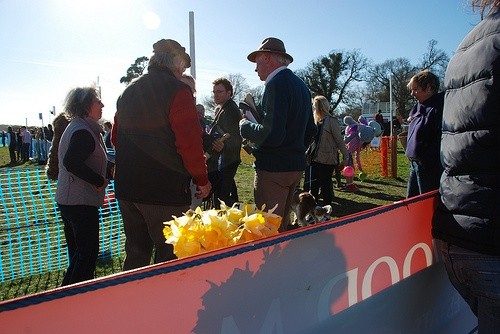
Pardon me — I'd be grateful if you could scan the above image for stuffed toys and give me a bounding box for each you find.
[343,115,382,172]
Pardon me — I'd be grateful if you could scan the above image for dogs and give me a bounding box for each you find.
[290,189,332,226]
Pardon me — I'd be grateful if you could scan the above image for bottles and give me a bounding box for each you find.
[205,133,230,164]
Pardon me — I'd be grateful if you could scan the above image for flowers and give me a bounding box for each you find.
[163,198,283,259]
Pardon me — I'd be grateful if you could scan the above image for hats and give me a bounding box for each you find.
[153,39,192,68]
[247,37,293,63]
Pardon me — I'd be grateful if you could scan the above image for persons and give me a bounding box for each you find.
[55,86,110,287]
[7,126,17,163]
[405,69,445,198]
[375,110,384,125]
[16,124,54,163]
[110,38,212,272]
[431,0,500,333]
[179,74,230,168]
[239,37,319,232]
[196,104,205,117]
[306,95,350,206]
[103,122,114,180]
[204,77,243,209]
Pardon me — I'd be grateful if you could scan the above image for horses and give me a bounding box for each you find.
[382,119,402,136]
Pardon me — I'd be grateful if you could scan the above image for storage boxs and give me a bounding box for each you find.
[398,131,408,151]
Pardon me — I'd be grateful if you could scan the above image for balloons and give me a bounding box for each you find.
[343,166,355,178]
[359,172,368,181]
